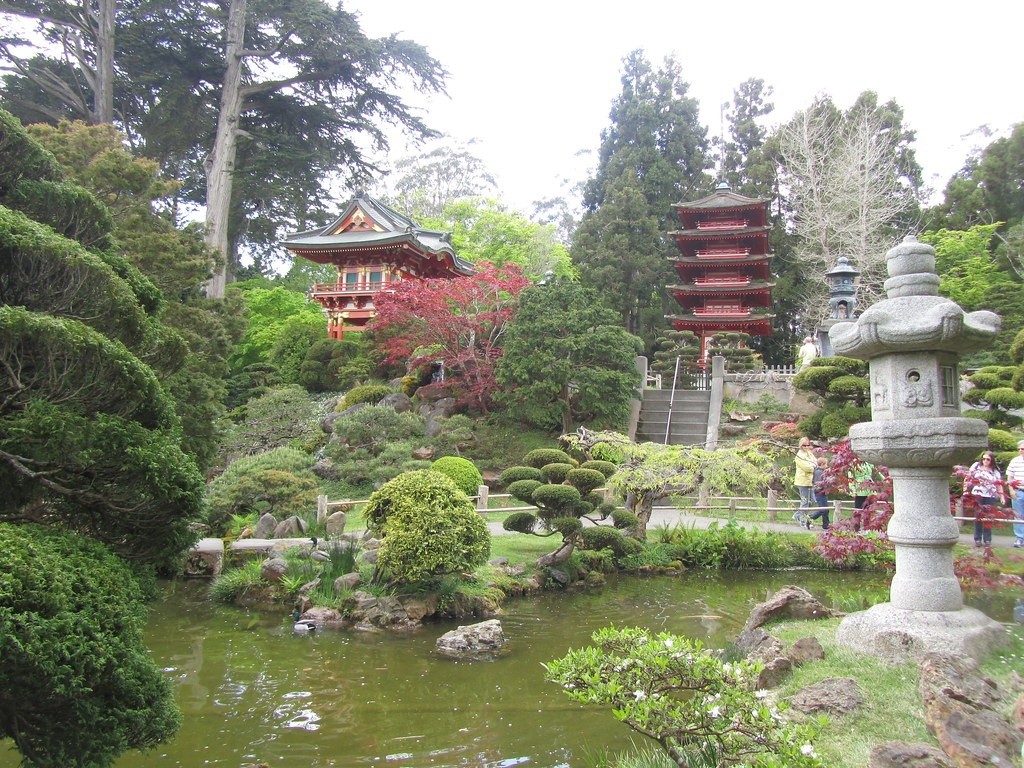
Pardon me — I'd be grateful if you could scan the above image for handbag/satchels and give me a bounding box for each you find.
[963,481,972,498]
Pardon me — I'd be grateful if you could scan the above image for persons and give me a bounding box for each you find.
[799,337,816,371]
[962,451,1005,547]
[804,458,831,530]
[1006,440,1024,548]
[848,459,888,532]
[792,437,817,528]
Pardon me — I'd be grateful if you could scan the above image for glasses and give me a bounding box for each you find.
[802,444,810,447]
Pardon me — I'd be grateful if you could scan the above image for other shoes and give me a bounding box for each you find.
[792,515,802,522]
[985,540,991,547]
[805,516,811,529]
[1014,539,1024,547]
[822,524,830,529]
[975,539,981,546]
[800,522,813,528]
